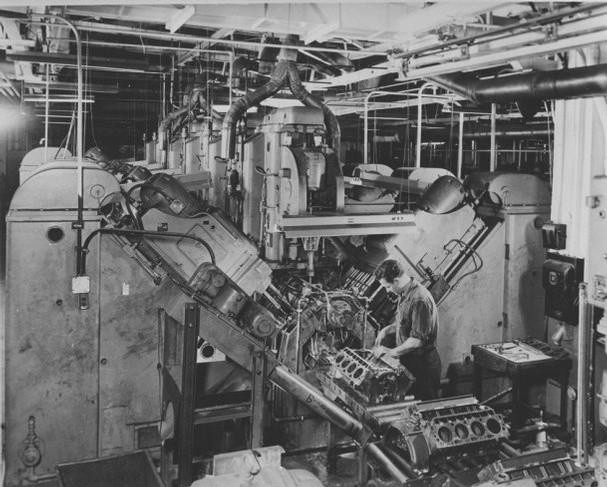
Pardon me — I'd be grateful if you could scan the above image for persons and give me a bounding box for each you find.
[372,259,444,400]
